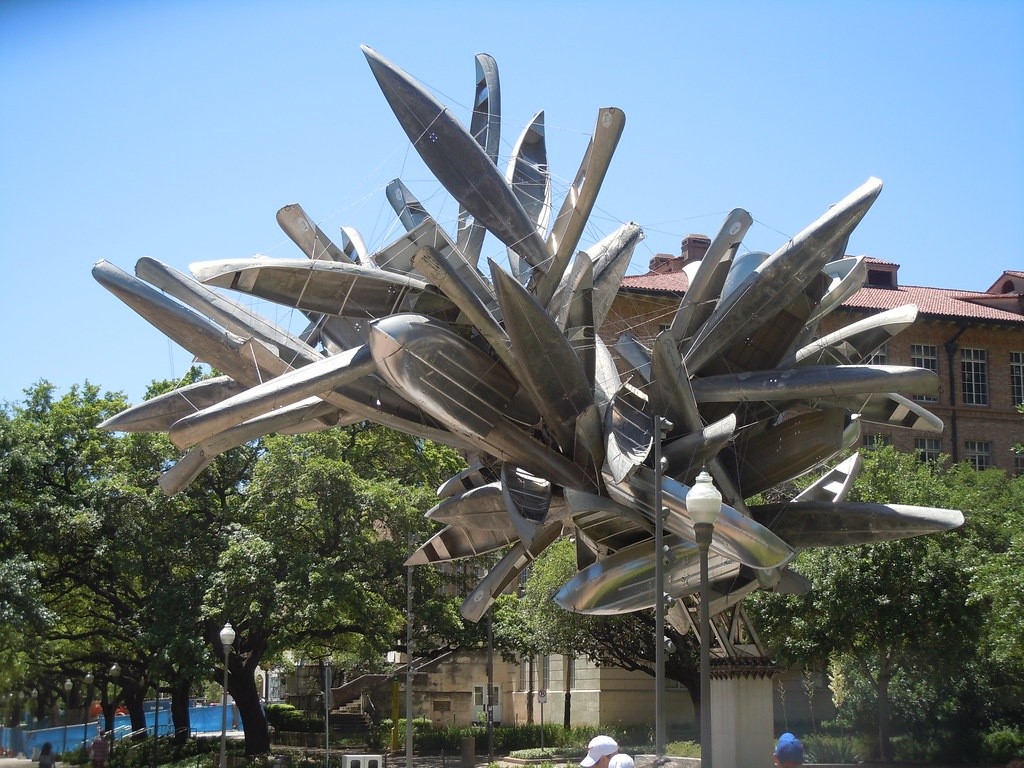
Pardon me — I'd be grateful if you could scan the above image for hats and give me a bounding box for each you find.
[608,754,635,768]
[580,735,618,767]
[776,733,804,762]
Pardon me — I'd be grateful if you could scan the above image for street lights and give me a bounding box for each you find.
[30,687,38,730]
[686,457,722,768]
[220,618,235,768]
[61,678,73,756]
[110,661,121,768]
[80,670,95,764]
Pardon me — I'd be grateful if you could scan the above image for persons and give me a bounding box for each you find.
[580,735,635,768]
[38,742,56,768]
[773,733,805,768]
[90,730,109,768]
